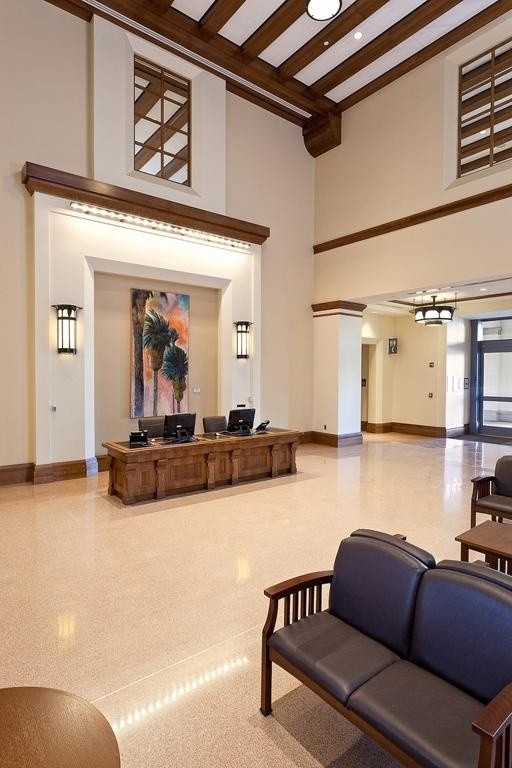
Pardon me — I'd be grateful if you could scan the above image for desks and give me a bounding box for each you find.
[0,685,124,767]
[454,516,512,574]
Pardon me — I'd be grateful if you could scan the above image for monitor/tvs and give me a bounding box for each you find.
[163,413,196,444]
[227,408,255,436]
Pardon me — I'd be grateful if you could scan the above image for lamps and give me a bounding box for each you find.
[302,1,345,23]
[408,292,461,329]
[232,320,253,360]
[50,302,84,355]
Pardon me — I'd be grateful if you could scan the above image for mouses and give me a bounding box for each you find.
[215,432,221,436]
[151,438,156,441]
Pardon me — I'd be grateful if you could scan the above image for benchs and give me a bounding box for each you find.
[349,527,510,588]
[469,455,512,528]
[252,528,512,767]
